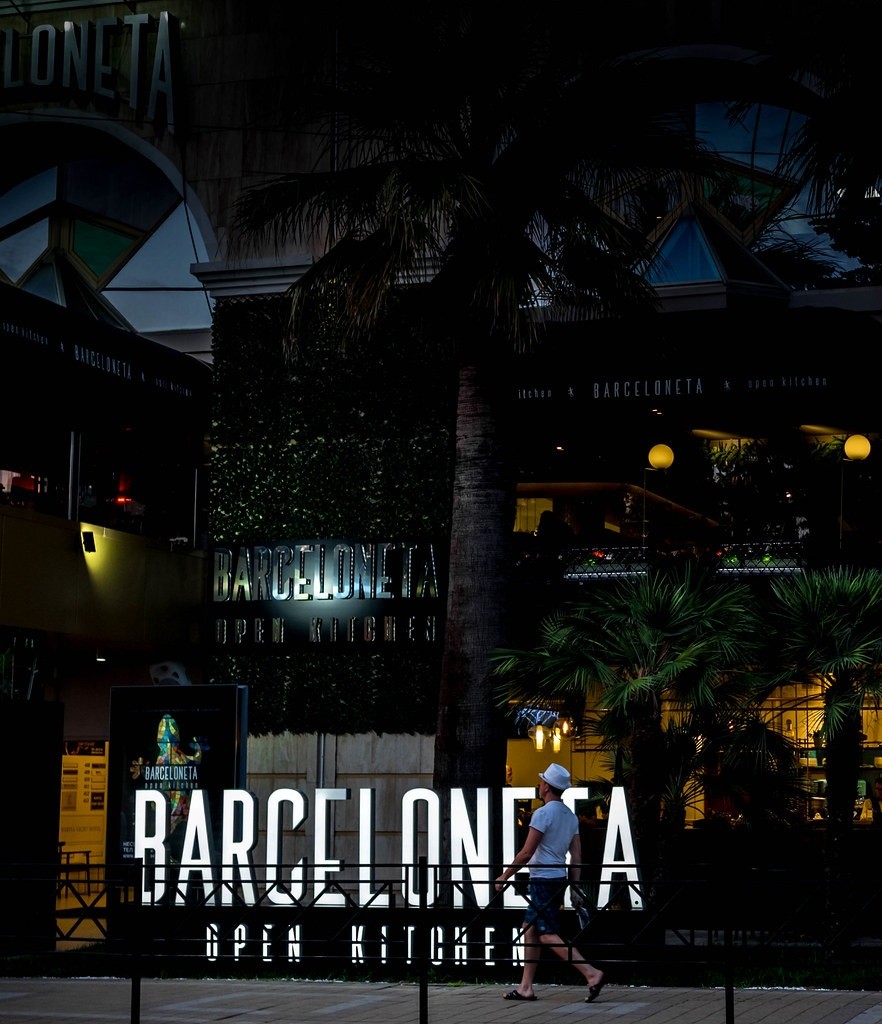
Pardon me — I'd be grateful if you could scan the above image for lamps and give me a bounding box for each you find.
[80,532,96,554]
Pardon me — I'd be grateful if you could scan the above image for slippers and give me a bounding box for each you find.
[585,966,610,1002]
[503,990,537,1001]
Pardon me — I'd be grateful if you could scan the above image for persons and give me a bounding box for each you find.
[493,763,606,1002]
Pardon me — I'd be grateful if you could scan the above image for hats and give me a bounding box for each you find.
[539,763,572,791]
